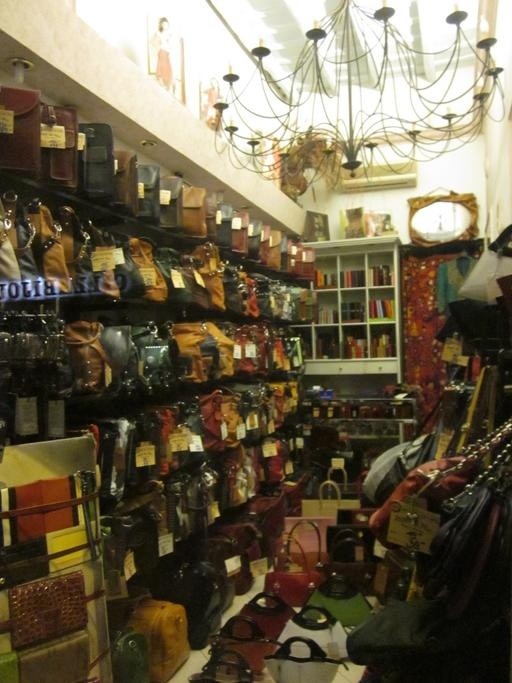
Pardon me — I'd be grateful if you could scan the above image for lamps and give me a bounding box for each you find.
[6,57,34,86]
[216,189,224,203]
[205,0,506,192]
[139,140,156,155]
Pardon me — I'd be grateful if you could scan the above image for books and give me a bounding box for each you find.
[317,266,396,357]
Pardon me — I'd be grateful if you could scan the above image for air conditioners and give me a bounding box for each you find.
[341,162,418,194]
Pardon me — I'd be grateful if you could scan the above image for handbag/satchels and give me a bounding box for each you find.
[205,422,347,553]
[0,469,104,590]
[324,554,376,597]
[188,649,270,683]
[180,182,210,240]
[262,568,322,609]
[303,386,411,421]
[238,591,297,643]
[75,119,116,201]
[0,325,313,443]
[0,650,20,683]
[35,102,80,191]
[5,571,94,650]
[0,84,42,179]
[0,200,316,324]
[263,637,343,683]
[17,627,91,683]
[208,614,278,675]
[106,146,141,218]
[95,444,218,573]
[133,162,166,224]
[303,571,377,627]
[276,602,351,662]
[155,176,186,229]
[348,419,512,683]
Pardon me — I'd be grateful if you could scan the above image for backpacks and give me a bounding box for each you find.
[109,625,152,683]
[199,533,255,596]
[124,597,193,683]
[169,559,230,649]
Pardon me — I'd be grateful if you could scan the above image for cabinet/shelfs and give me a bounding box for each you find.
[301,397,415,445]
[281,237,402,383]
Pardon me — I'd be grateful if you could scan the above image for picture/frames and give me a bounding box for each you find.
[407,192,479,247]
